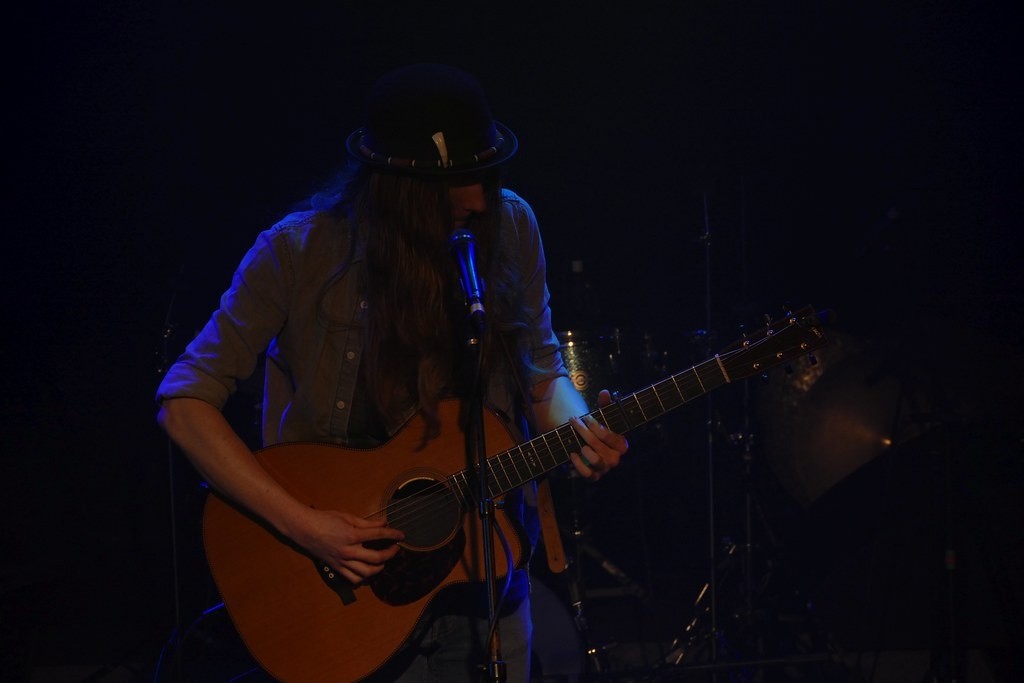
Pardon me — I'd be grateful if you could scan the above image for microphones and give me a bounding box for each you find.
[448,229,486,332]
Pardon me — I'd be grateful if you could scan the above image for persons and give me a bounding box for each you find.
[155,64,627,683]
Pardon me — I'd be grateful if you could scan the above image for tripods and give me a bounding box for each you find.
[653,386,753,683]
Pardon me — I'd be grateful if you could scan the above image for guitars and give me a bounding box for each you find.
[201,302,833,683]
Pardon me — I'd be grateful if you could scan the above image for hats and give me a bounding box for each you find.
[344,63,519,177]
